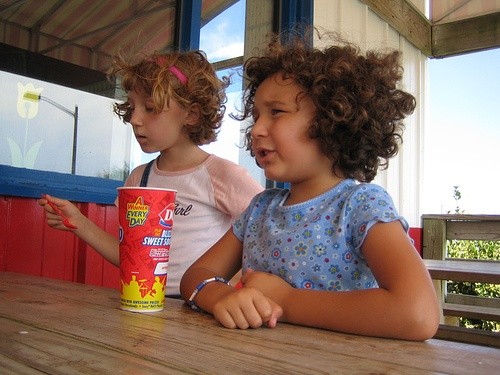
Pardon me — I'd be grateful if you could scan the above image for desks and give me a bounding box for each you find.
[0,270,500,375]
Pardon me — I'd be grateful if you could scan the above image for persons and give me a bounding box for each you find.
[178,30,440,342]
[37,47,264,298]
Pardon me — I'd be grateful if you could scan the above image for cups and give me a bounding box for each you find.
[118,187,177,312]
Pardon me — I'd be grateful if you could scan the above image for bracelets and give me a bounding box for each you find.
[188,276,232,313]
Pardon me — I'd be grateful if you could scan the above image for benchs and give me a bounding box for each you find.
[421,258,500,350]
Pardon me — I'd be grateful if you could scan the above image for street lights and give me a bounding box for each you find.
[23,92,78,174]
